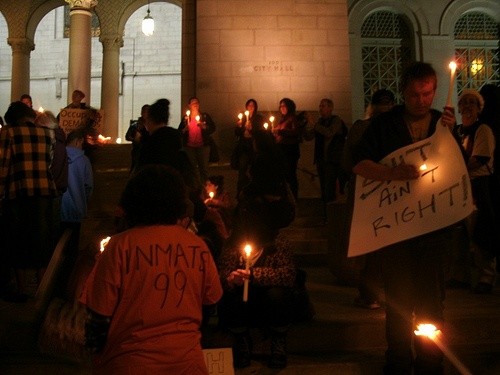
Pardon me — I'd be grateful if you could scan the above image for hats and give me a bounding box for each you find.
[148,99,169,124]
[371,88,395,105]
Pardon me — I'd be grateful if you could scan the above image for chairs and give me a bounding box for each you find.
[0,228,72,323]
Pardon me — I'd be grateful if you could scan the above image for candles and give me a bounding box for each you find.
[245,110,249,122]
[186,110,190,125]
[243,243,252,301]
[238,113,243,127]
[270,116,275,130]
[414,324,471,375]
[446,61,457,107]
